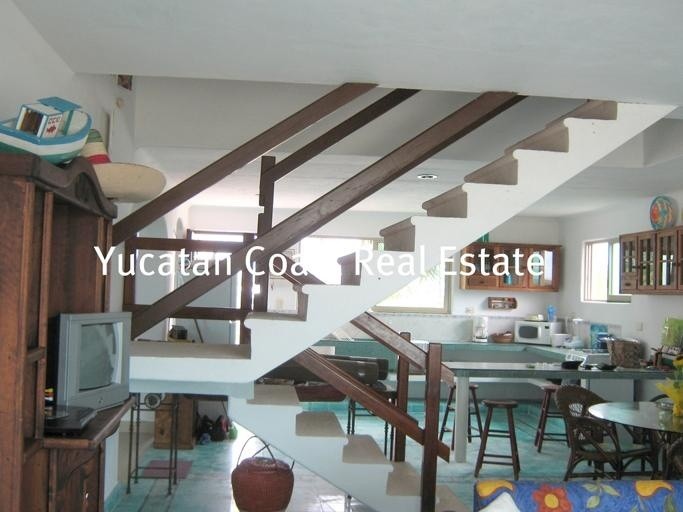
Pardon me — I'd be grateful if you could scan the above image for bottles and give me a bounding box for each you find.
[655,345,662,371]
[597,332,609,353]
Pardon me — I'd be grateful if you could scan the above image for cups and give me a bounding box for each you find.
[504,274,512,285]
[547,307,557,322]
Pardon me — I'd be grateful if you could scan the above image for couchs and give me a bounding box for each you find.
[474,480,683,512]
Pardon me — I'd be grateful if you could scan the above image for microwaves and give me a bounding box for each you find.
[514,321,563,345]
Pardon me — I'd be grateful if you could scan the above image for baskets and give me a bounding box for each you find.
[491,333,513,342]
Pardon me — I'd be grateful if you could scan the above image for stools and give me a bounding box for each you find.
[474,400,520,480]
[347,392,397,499]
[439,383,483,450]
[534,384,571,453]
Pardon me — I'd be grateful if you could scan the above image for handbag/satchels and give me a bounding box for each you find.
[606,335,642,368]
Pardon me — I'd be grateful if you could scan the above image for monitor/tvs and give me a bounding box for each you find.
[45,311,131,421]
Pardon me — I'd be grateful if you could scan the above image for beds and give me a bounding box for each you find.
[587,401,682,479]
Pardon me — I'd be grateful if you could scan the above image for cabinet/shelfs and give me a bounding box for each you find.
[459,243,562,292]
[0,152,137,512]
[620,226,683,295]
[128,392,180,495]
[153,394,196,449]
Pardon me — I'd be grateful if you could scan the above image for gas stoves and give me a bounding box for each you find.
[565,348,611,367]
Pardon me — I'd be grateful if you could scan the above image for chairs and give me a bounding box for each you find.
[641,394,683,475]
[554,384,658,479]
[650,436,683,479]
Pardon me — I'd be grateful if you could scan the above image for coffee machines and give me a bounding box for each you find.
[472,316,488,343]
[564,318,585,349]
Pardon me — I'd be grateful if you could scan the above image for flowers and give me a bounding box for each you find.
[655,359,683,403]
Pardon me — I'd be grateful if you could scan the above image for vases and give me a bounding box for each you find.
[673,382,683,416]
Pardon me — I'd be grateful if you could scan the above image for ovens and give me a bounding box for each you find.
[564,360,634,416]
